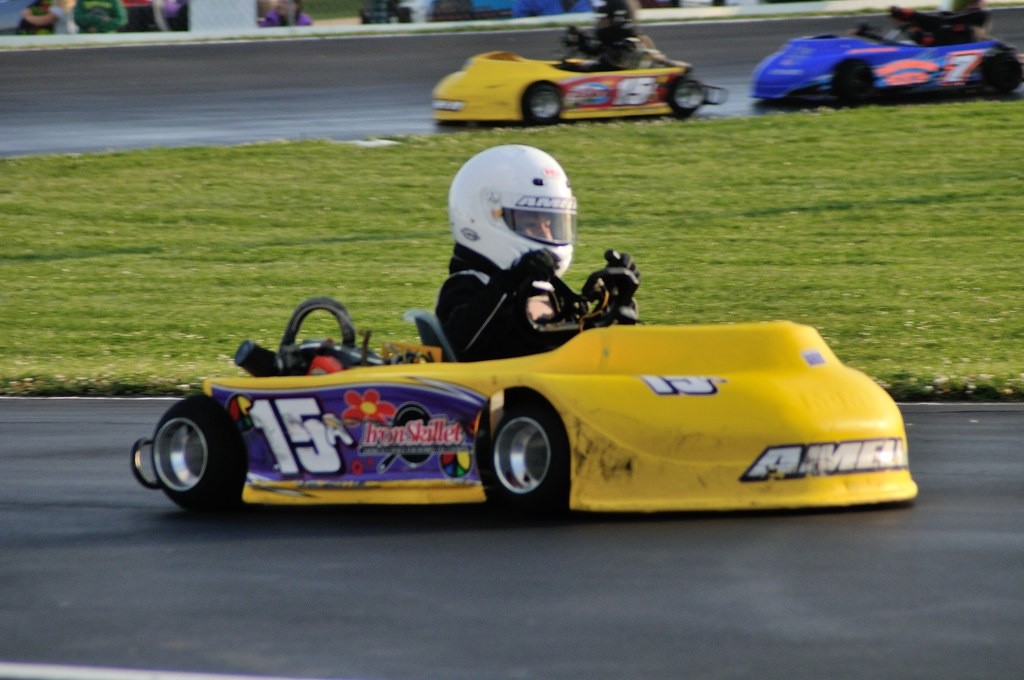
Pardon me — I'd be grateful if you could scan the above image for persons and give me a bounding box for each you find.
[891,0,988,46]
[564,0,641,70]
[436,145,640,362]
[15,0,189,35]
[258,0,311,26]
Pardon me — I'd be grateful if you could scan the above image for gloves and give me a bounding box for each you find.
[604,248,641,280]
[508,248,561,285]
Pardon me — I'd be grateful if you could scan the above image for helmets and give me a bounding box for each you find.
[449,144,577,279]
[591,0,636,29]
